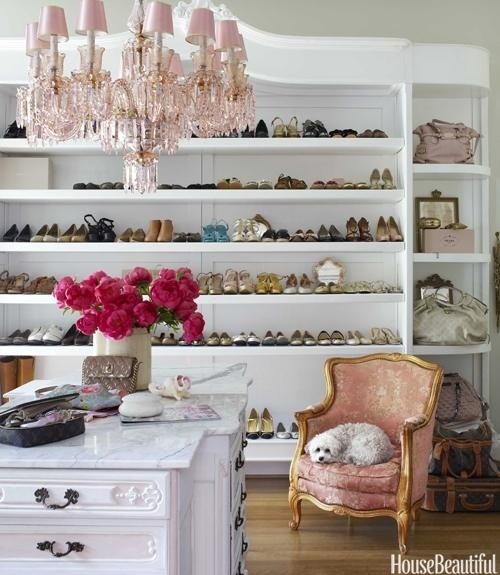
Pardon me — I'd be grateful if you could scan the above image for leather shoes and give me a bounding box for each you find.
[289,330,315,346]
[61,323,92,346]
[245,406,275,439]
[1,328,30,345]
[375,212,403,243]
[151,331,177,346]
[178,334,206,347]
[0,213,371,242]
[261,330,289,346]
[346,329,371,346]
[29,323,60,344]
[234,330,260,346]
[2,120,27,138]
[276,421,300,438]
[208,332,232,346]
[317,330,344,345]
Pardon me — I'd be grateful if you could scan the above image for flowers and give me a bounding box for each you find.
[52,265,204,351]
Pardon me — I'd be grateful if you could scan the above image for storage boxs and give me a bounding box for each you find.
[420,229,475,254]
[0,157,53,190]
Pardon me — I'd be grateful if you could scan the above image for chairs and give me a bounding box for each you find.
[432,370,496,478]
[284,352,444,552]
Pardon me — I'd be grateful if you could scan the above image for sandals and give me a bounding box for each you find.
[191,114,399,138]
[195,267,314,295]
[372,326,401,346]
[0,268,58,295]
[316,276,404,294]
[70,167,402,192]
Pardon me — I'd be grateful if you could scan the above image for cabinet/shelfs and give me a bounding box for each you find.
[1,380,252,575]
[0,424,207,575]
[409,38,497,454]
[0,0,412,475]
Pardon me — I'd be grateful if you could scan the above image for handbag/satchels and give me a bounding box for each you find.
[434,370,489,431]
[80,355,140,399]
[413,278,489,349]
[427,422,493,479]
[412,118,482,165]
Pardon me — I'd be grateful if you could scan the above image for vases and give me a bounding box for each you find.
[94,328,153,393]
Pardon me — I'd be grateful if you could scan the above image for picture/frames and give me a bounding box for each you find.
[416,196,460,253]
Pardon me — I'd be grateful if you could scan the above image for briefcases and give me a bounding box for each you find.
[419,472,500,514]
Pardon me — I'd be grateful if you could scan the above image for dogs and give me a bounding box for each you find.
[303,421,394,465]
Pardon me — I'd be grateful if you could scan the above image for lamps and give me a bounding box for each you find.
[13,0,259,195]
